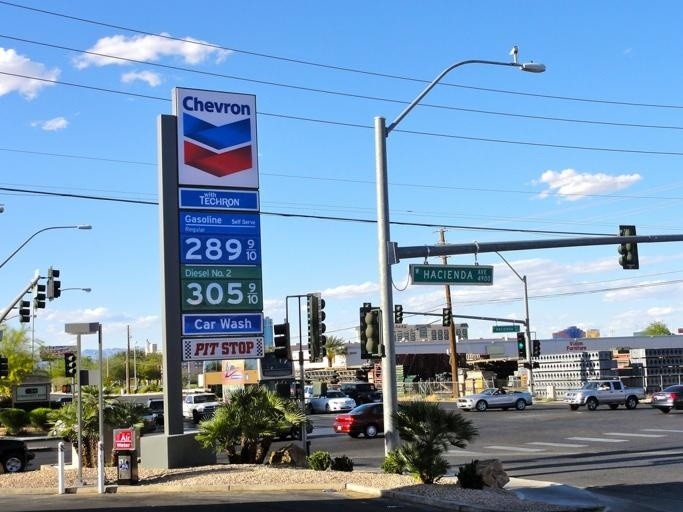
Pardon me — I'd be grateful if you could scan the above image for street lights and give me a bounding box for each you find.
[374,45,546,458]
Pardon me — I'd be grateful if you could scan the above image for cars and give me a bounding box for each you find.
[651,384,682,413]
[307,390,356,413]
[457,387,532,411]
[146,397,163,425]
[332,402,383,439]
[182,391,226,423]
[0,438,36,473]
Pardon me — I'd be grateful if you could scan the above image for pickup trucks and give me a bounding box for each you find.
[341,380,381,406]
[567,379,645,411]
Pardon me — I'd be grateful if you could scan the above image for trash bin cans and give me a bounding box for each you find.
[547,385,555,401]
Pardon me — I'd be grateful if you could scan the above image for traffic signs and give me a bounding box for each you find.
[492,326,519,332]
[408,264,492,285]
[176,87,266,362]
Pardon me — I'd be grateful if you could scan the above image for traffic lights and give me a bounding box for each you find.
[62,385,72,393]
[516,331,526,359]
[360,303,386,359]
[442,308,451,326]
[0,356,9,375]
[532,340,540,357]
[616,225,638,270]
[48,267,61,299]
[19,299,31,323]
[32,283,46,309]
[307,292,327,363]
[273,324,289,358]
[395,304,403,324]
[65,353,77,377]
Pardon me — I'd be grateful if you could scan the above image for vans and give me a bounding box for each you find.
[303,385,314,405]
